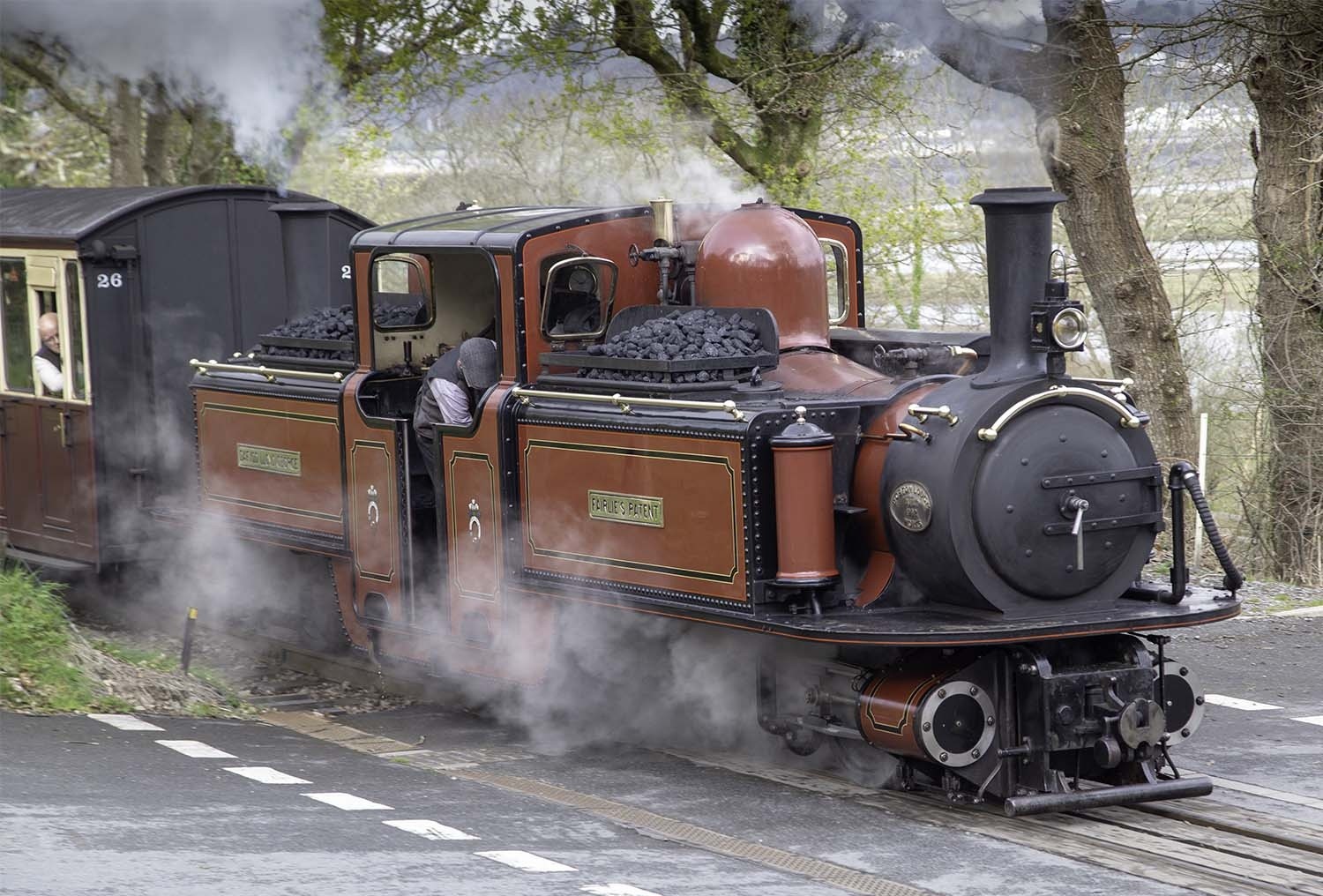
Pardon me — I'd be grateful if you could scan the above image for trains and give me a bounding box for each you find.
[0,178,1243,815]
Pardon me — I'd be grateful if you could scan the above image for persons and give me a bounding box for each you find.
[34,312,64,398]
[413,337,497,485]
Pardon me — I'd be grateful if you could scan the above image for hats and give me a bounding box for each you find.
[459,337,500,389]
